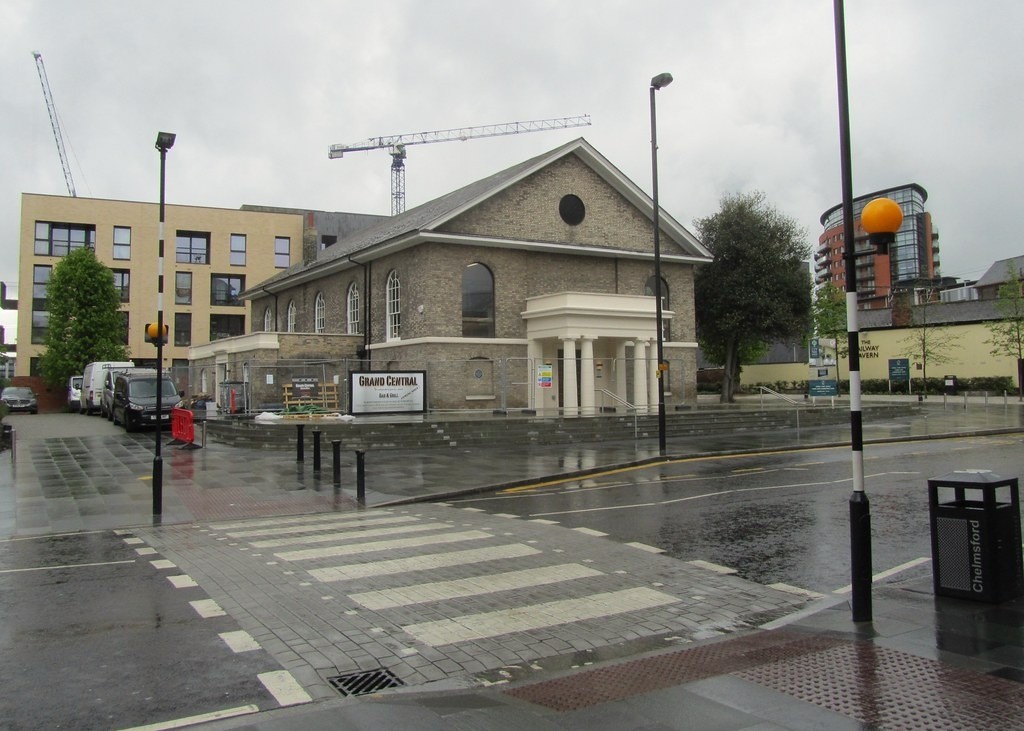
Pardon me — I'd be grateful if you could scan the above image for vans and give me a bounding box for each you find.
[80,360,136,416]
[112,371,184,434]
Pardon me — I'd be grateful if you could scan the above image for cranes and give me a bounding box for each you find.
[324,113,593,215]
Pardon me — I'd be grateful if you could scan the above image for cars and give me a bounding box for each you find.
[101,368,127,420]
[1,386,40,414]
[68,375,84,411]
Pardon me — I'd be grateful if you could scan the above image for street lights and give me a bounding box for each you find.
[647,71,679,458]
[147,129,178,528]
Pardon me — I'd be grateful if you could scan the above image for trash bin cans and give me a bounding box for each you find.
[219,381,226,414]
[928,469,1023,609]
[223,380,249,414]
